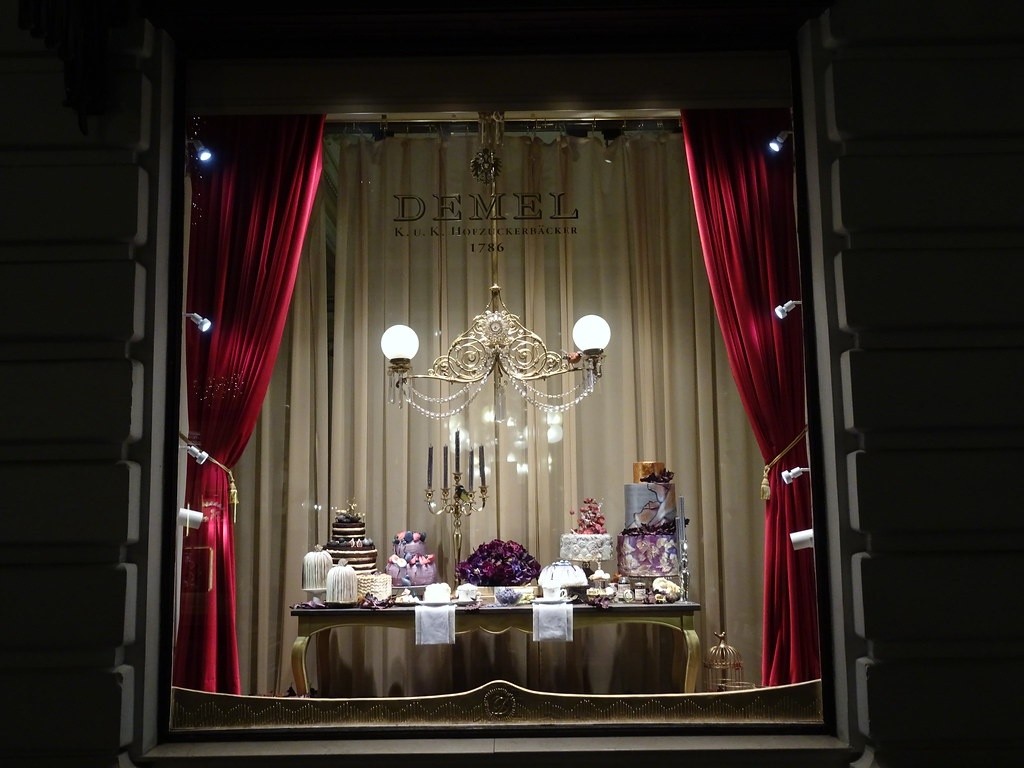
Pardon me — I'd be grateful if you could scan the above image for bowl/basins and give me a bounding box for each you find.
[494,590,523,607]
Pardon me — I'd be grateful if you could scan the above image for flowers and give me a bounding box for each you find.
[454,537,543,588]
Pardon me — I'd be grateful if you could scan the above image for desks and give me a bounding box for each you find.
[290,598,700,700]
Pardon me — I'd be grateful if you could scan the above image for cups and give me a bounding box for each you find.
[424,582,451,603]
[457,583,481,601]
[542,581,567,600]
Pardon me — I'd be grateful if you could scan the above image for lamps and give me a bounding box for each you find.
[175,115,210,526]
[379,98,611,420]
[769,127,817,552]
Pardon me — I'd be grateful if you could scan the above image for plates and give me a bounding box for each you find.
[416,601,454,606]
[531,598,572,603]
[454,601,480,605]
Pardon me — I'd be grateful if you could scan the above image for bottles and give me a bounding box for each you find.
[618,576,630,601]
[634,582,647,601]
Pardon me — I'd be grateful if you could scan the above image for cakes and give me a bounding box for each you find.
[616,460,682,576]
[301,498,613,604]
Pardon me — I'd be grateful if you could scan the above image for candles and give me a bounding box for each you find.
[468,447,474,491]
[479,443,486,484]
[444,444,448,488]
[428,444,433,489]
[454,428,460,472]
